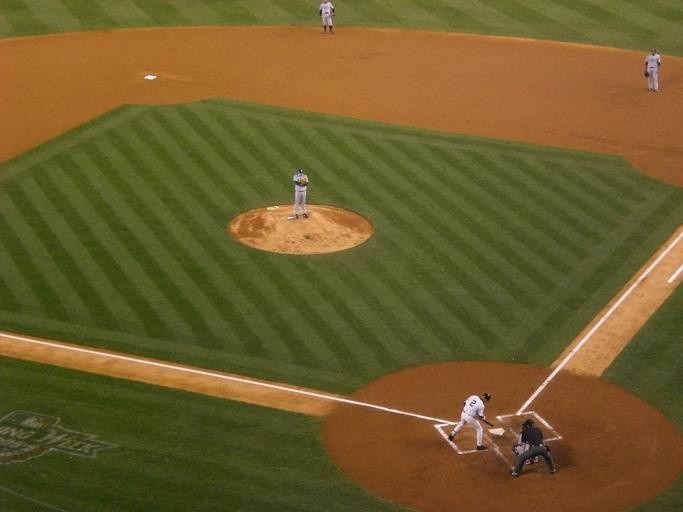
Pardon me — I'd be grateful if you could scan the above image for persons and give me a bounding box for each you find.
[319,0,336,33]
[513,425,539,465]
[293,169,308,220]
[449,392,491,450]
[512,419,557,477]
[644,48,661,92]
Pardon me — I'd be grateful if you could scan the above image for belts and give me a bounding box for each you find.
[530,444,543,447]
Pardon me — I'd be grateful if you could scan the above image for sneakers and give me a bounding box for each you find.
[296,213,307,220]
[449,434,453,440]
[478,445,487,451]
[510,470,517,476]
[551,468,559,473]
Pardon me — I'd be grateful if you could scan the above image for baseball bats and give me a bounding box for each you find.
[476,414,494,427]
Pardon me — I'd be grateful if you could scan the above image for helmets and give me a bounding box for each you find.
[482,393,490,401]
[523,419,534,430]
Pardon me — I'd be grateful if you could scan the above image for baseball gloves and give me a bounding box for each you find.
[331,13,336,16]
[299,178,305,184]
[645,71,649,76]
